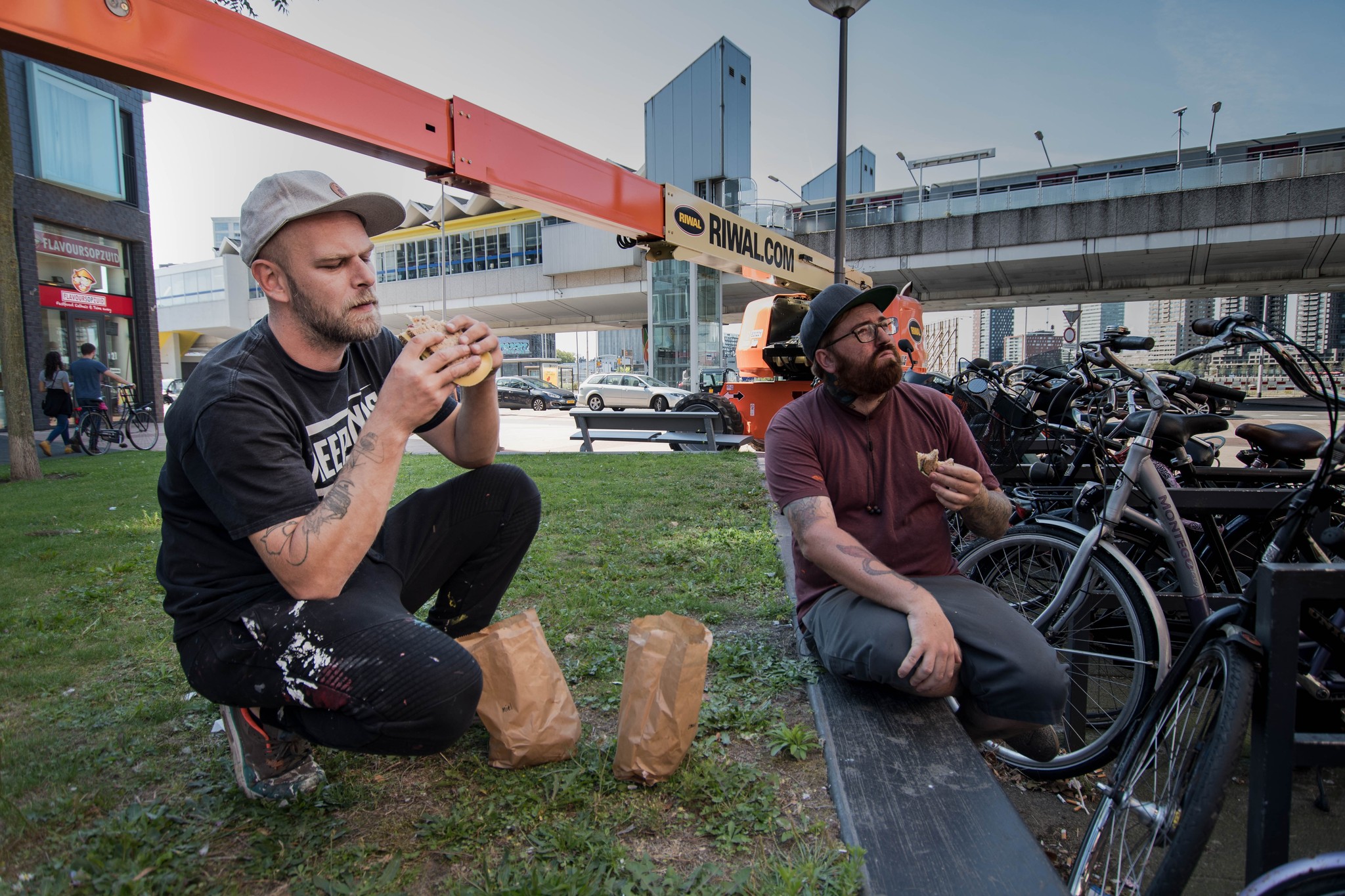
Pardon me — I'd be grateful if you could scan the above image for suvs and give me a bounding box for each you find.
[496,375,577,411]
[577,372,695,412]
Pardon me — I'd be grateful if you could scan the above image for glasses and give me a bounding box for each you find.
[824,316,899,347]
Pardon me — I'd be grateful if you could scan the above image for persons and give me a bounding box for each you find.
[764,284,1069,764]
[69,343,136,454]
[157,170,541,804]
[38,352,74,455]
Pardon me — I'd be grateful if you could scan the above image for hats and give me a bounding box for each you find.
[800,283,898,359]
[238,171,408,266]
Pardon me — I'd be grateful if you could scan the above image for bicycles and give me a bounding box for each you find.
[72,384,159,456]
[897,312,1345,896]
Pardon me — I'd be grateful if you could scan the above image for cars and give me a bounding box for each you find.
[161,378,187,403]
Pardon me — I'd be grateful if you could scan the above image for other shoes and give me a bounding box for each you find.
[39,441,52,456]
[221,702,329,802]
[1005,724,1060,763]
[69,436,82,453]
[65,447,75,454]
[88,446,104,453]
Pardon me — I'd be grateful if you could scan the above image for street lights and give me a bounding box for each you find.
[809,0,870,285]
[619,320,630,373]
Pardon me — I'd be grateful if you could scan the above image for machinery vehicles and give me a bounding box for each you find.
[0,0,1002,460]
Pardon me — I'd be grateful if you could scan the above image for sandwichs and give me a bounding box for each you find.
[398,315,493,388]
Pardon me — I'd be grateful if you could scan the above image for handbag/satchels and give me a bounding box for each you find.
[42,399,45,409]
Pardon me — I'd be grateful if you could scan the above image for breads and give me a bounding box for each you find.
[916,448,954,477]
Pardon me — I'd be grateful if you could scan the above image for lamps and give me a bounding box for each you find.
[1208,101,1222,151]
[1035,131,1052,168]
[768,175,810,205]
[896,152,918,186]
[618,321,629,327]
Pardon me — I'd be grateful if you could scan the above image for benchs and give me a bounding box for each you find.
[569,411,754,451]
[420,267,439,277]
